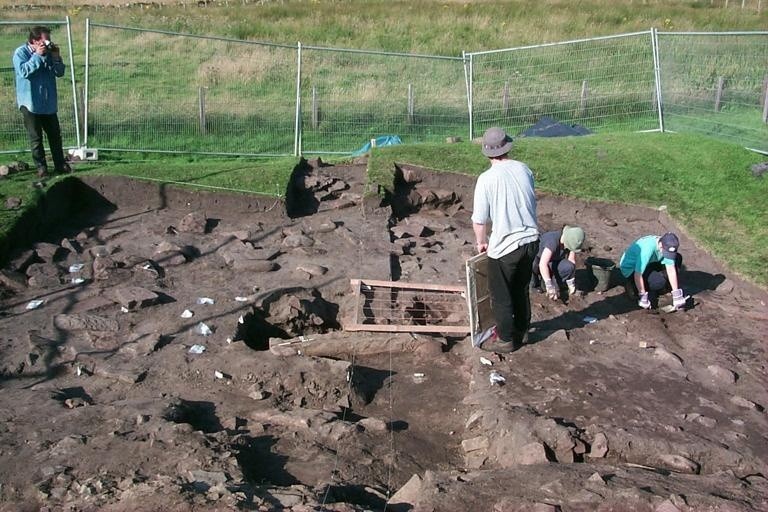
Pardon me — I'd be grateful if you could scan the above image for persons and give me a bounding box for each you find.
[13,26,70,178]
[472,126,540,353]
[531,226,586,301]
[618,233,686,313]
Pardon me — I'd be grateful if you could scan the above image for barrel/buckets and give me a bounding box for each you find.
[583,256,616,292]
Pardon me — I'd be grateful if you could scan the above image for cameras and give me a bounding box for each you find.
[43,40,55,50]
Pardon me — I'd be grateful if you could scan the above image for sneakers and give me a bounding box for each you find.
[532,282,543,294]
[55,163,71,173]
[625,280,639,301]
[35,167,47,177]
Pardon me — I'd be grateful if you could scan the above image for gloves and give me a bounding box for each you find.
[544,279,558,301]
[671,289,686,307]
[565,277,577,296]
[637,291,651,309]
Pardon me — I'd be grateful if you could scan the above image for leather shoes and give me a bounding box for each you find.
[517,329,529,344]
[481,327,514,354]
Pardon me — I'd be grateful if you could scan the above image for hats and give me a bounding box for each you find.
[562,225,585,253]
[481,126,514,157]
[661,232,679,259]
